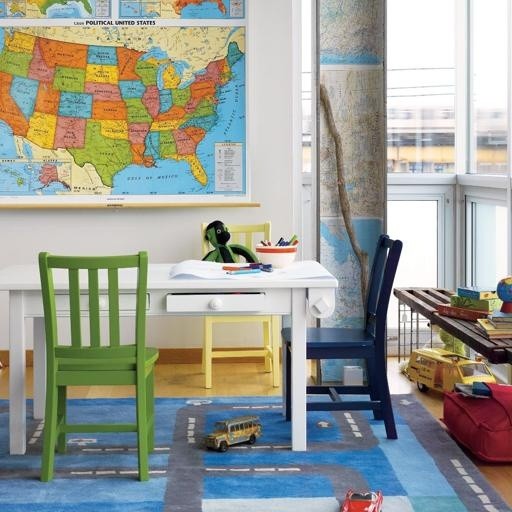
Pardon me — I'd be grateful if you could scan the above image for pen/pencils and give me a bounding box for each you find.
[224,267,260,274]
[261,235,298,246]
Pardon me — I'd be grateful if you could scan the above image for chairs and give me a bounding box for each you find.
[38,251,160,482]
[281,233,403,440]
[201,222,281,389]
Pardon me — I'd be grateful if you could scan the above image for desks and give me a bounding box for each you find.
[0,260,338,456]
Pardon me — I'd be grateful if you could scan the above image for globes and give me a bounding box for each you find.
[497,277,512,313]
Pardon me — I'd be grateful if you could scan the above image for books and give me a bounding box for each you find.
[436,285,512,339]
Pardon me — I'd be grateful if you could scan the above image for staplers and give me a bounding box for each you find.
[251,263,273,272]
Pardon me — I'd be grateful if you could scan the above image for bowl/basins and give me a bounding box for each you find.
[256,246,297,269]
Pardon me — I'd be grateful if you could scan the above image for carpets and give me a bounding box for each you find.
[0,393,512,512]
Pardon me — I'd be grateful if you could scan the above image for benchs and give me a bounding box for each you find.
[393,287,512,363]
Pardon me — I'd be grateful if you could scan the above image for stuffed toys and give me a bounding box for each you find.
[202,218,260,262]
